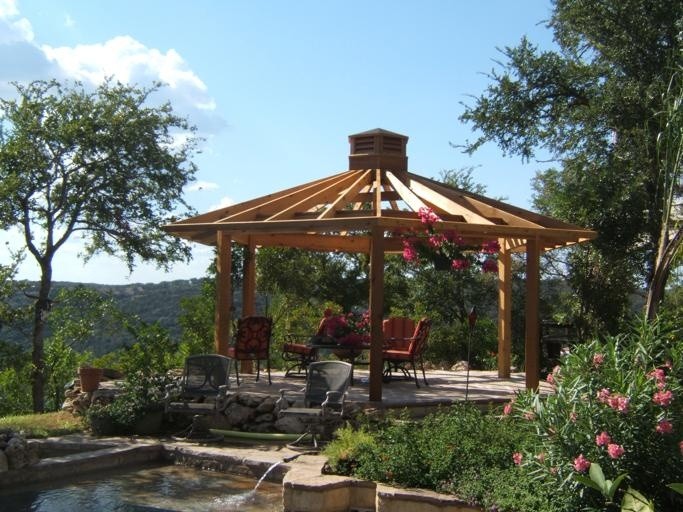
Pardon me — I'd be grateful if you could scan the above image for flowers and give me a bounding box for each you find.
[318,307,372,344]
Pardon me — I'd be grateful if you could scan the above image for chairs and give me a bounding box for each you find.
[283,333,317,378]
[276,360,353,451]
[163,353,232,443]
[381,316,431,388]
[229,315,273,386]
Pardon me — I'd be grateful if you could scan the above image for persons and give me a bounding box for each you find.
[315,306,333,336]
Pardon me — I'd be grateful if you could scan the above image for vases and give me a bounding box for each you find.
[77,367,104,392]
[333,346,363,358]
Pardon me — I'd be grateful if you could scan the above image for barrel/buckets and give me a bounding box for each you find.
[78,362,103,392]
